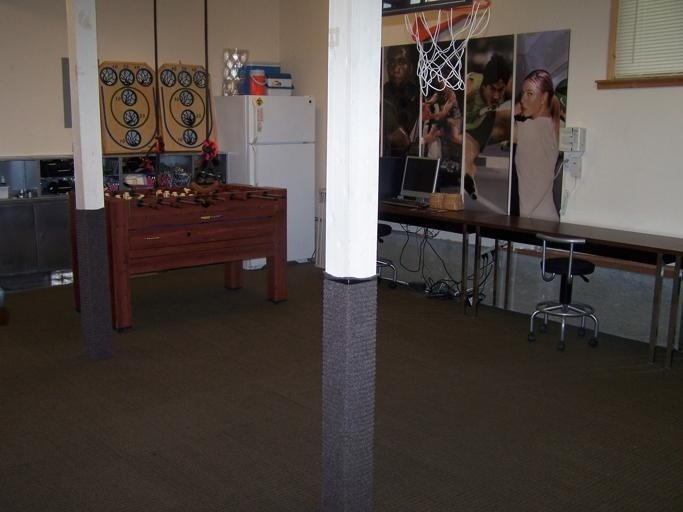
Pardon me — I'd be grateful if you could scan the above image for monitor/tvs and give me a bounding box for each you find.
[400,156,440,207]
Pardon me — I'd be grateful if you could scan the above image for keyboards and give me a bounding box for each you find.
[381,198,429,210]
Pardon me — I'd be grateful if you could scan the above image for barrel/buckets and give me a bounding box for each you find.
[249,68,268,95]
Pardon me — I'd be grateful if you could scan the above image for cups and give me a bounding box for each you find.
[223,80,240,96]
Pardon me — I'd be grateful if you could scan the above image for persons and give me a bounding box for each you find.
[383,46,419,145]
[513,69,566,222]
[422,72,463,147]
[464,54,512,200]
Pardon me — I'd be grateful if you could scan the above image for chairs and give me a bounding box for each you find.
[378,225,396,286]
[529,233,598,347]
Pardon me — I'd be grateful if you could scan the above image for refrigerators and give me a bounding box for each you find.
[217,94,317,270]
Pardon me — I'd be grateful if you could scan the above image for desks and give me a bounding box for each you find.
[474,214,681,372]
[378,202,500,315]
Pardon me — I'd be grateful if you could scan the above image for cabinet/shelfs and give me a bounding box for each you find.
[0,154,228,294]
